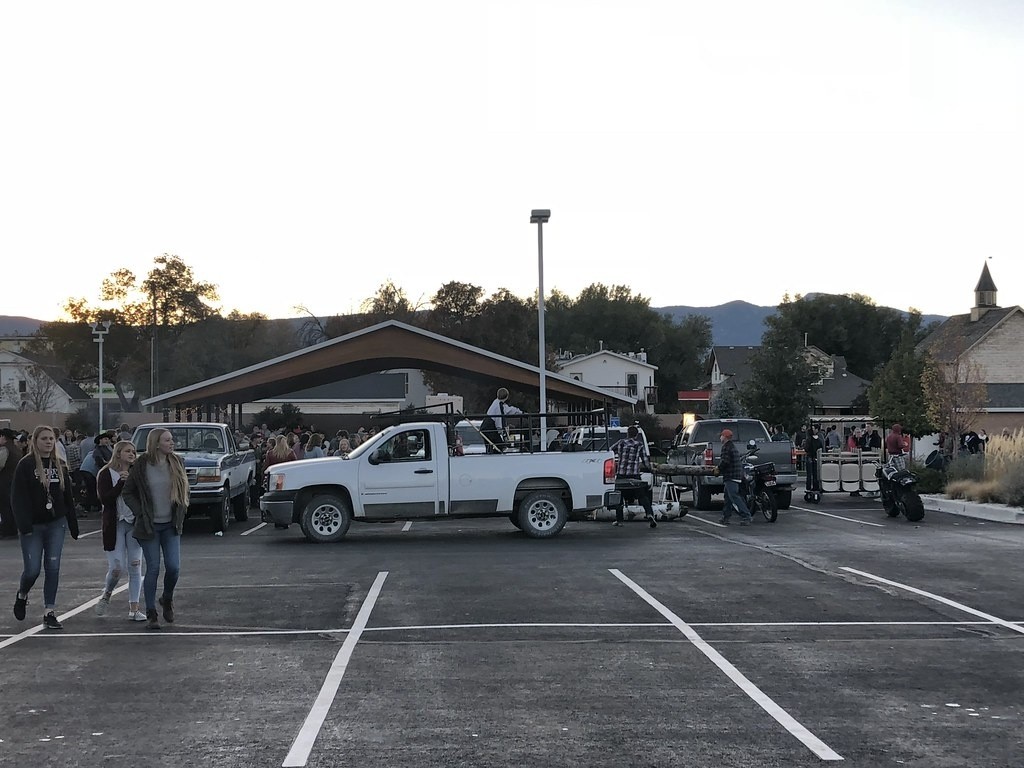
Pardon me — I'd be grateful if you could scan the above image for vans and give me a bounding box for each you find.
[568,423,650,464]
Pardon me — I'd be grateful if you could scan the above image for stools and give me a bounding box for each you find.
[659,482,679,505]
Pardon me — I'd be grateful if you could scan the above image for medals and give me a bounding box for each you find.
[46,503,52,509]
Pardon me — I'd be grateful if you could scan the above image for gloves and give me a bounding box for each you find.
[712,465,721,477]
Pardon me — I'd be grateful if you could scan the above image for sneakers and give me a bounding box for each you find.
[96,597,109,617]
[146,609,161,630]
[128,611,147,621]
[13,588,27,621]
[43,610,61,629]
[159,592,174,623]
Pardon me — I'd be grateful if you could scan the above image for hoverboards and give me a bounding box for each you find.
[803,458,821,504]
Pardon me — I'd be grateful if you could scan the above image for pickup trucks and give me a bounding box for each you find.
[258,422,648,543]
[130,422,256,532]
[657,418,798,511]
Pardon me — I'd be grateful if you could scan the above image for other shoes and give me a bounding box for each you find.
[647,514,657,529]
[613,521,623,527]
[720,516,732,525]
[741,519,751,526]
[76,512,88,519]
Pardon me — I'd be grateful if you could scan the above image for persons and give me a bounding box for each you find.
[804,424,825,490]
[764,421,789,441]
[795,423,881,471]
[10,427,80,628]
[480,387,527,454]
[0,424,137,539]
[123,428,191,629]
[1002,426,1016,442]
[563,425,574,443]
[932,432,953,454]
[454,429,463,453]
[95,441,146,620]
[712,430,753,526]
[961,425,989,455]
[612,426,658,528]
[887,424,908,471]
[234,423,380,507]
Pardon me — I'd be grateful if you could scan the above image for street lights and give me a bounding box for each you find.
[88,320,112,435]
[528,209,554,453]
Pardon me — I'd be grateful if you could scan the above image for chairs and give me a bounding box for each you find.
[816,447,884,491]
[204,439,219,449]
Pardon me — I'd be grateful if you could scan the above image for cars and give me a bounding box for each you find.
[453,420,492,455]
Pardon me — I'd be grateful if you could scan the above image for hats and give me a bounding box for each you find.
[251,434,261,441]
[0,428,18,440]
[94,430,112,444]
[717,430,733,438]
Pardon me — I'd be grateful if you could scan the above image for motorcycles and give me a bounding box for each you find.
[863,452,925,523]
[737,440,779,523]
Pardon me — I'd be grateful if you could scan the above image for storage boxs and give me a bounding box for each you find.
[754,462,775,477]
